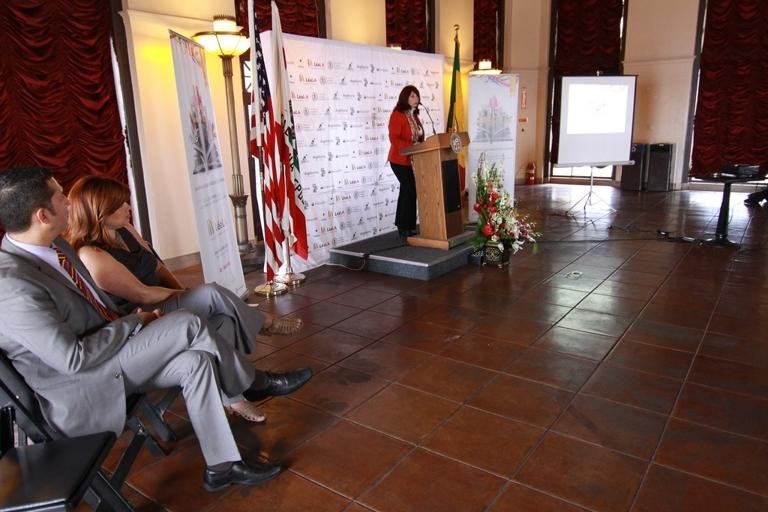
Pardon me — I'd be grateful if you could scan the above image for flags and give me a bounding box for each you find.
[267,1,309,265]
[248,1,299,282]
[444,35,468,200]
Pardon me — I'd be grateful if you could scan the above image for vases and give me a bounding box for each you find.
[481,238,511,269]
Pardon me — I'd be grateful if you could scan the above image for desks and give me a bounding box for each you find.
[686,173,767,249]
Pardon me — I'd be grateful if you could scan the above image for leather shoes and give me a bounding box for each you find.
[200,456,286,492]
[242,363,313,402]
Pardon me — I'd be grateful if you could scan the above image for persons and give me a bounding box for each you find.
[63,175,305,424]
[0,166,313,495]
[386,84,424,240]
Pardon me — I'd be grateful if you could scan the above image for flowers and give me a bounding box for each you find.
[466,153,543,256]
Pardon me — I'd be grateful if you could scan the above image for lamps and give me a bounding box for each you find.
[467,59,503,77]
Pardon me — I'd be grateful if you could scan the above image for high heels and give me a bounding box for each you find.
[225,398,268,423]
[257,316,305,338]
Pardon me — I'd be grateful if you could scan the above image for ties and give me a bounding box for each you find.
[53,246,122,325]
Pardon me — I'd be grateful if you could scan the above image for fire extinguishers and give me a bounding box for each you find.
[526,160,536,185]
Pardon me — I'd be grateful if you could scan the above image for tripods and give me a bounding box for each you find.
[565,167,618,215]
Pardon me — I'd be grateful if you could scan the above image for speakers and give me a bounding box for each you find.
[647,143,672,191]
[620,143,646,191]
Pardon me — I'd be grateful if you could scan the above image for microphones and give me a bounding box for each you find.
[417,101,438,134]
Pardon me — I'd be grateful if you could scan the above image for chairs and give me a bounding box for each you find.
[1,345,180,512]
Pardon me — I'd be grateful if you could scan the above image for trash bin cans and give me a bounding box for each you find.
[620,143,673,192]
